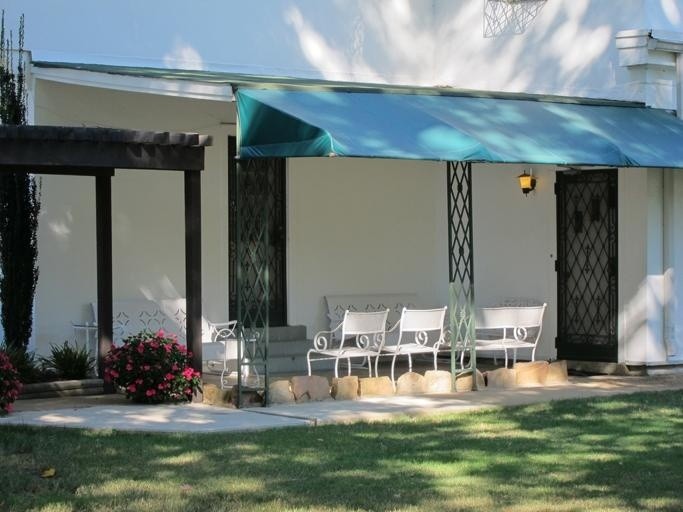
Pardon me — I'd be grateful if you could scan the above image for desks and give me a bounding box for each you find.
[73,326,99,377]
[216,331,260,390]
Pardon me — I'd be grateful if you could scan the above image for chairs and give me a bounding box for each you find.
[306,308,390,377]
[368,306,448,394]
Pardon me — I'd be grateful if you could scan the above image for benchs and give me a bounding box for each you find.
[90,297,244,379]
[432,302,547,371]
[324,293,417,370]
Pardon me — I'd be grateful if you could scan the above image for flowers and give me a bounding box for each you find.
[0,351,23,419]
[101,328,208,404]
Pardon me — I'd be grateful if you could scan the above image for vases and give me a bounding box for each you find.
[131,396,165,403]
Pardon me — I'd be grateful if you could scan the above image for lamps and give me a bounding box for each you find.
[518,169,537,197]
